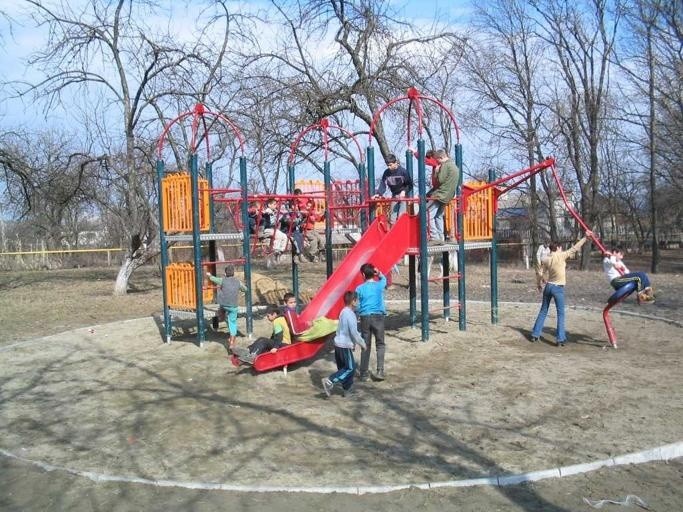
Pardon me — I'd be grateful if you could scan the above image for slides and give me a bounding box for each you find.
[232,212,421,371]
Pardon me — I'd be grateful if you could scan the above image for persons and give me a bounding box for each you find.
[601,247,656,305]
[371,153,413,229]
[408,147,442,202]
[353,262,387,383]
[535,239,551,293]
[205,265,248,352]
[247,188,327,270]
[229,304,291,365]
[282,292,313,337]
[321,290,368,397]
[425,149,460,247]
[528,229,594,346]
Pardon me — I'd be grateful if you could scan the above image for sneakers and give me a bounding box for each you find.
[638,287,663,305]
[229,346,255,364]
[271,253,281,266]
[360,369,385,381]
[321,377,334,396]
[530,334,543,342]
[228,335,236,345]
[309,252,326,263]
[428,238,444,246]
[344,389,364,398]
[557,339,567,347]
[293,255,308,264]
